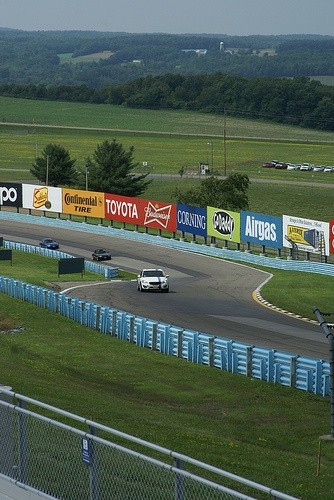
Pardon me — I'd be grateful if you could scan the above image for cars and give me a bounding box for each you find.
[136,268,169,292]
[262,159,334,169]
[92,248,111,260]
[39,238,59,249]
